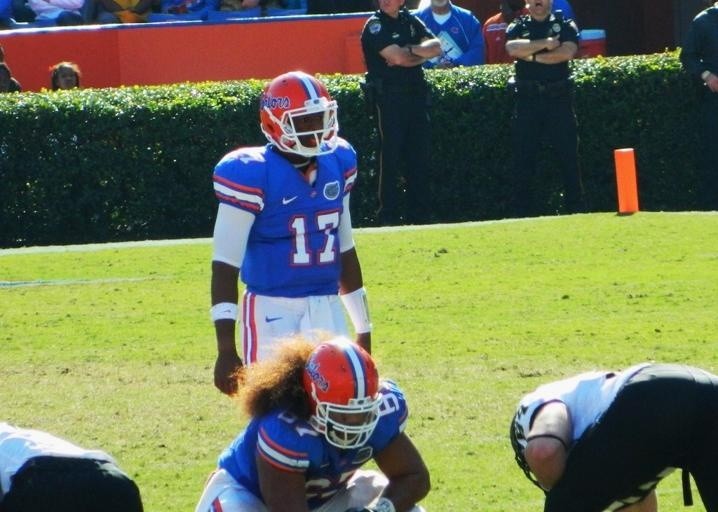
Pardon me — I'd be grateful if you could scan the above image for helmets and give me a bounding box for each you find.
[510,416,529,472]
[259,71,339,158]
[302,338,382,449]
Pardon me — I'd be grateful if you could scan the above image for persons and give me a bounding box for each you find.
[361,1,443,225]
[1,0,307,29]
[510,362,718,512]
[0,46,80,92]
[195,336,430,512]
[409,0,577,70]
[0,422,144,512]
[680,0,718,209]
[505,0,578,218]
[210,71,372,396]
